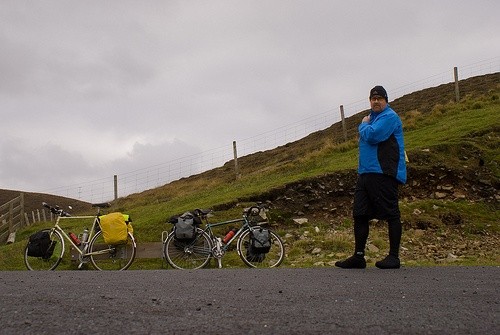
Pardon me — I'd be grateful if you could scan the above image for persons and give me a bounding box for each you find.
[335,85,407,269]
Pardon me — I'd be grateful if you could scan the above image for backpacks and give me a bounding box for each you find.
[173,211,198,243]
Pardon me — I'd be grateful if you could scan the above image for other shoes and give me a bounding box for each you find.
[375,255,401,269]
[335,252,366,269]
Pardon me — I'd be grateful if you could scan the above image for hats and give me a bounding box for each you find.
[368,86,388,104]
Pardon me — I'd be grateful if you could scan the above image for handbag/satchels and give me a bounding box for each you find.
[250,229,272,251]
[244,241,266,262]
[27,232,56,257]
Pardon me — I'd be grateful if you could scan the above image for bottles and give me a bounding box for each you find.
[217,236,223,246]
[68,232,81,246]
[82,228,89,245]
[223,227,235,243]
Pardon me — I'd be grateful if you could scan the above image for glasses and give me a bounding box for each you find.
[370,97,384,100]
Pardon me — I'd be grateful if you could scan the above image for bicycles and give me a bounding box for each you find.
[23,200,138,271]
[162,204,286,270]
